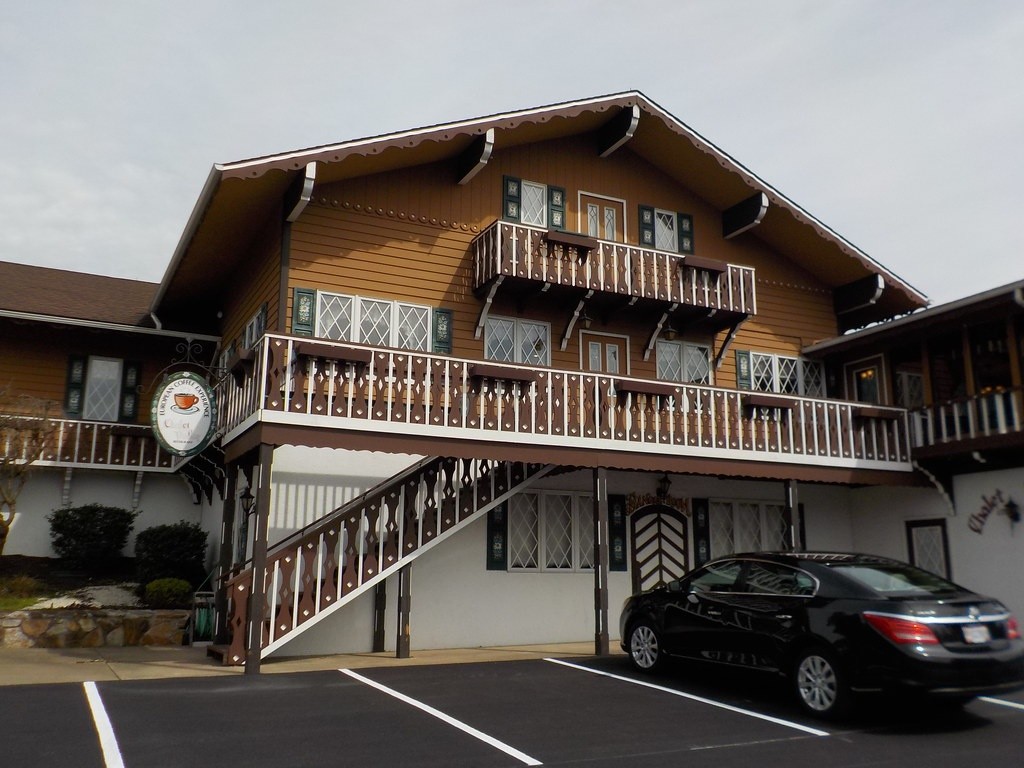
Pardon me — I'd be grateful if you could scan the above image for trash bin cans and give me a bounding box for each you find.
[193,592,215,646]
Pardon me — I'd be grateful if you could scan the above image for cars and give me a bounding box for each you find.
[616,550,1023,724]
